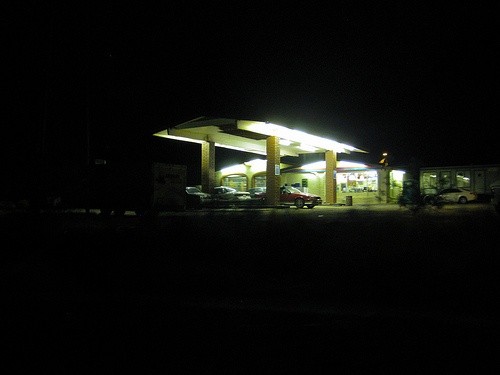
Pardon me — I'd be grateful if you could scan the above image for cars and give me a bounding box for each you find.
[260,186,323,209]
[244,187,266,197]
[434,187,477,204]
[213,184,251,202]
[182,185,211,206]
